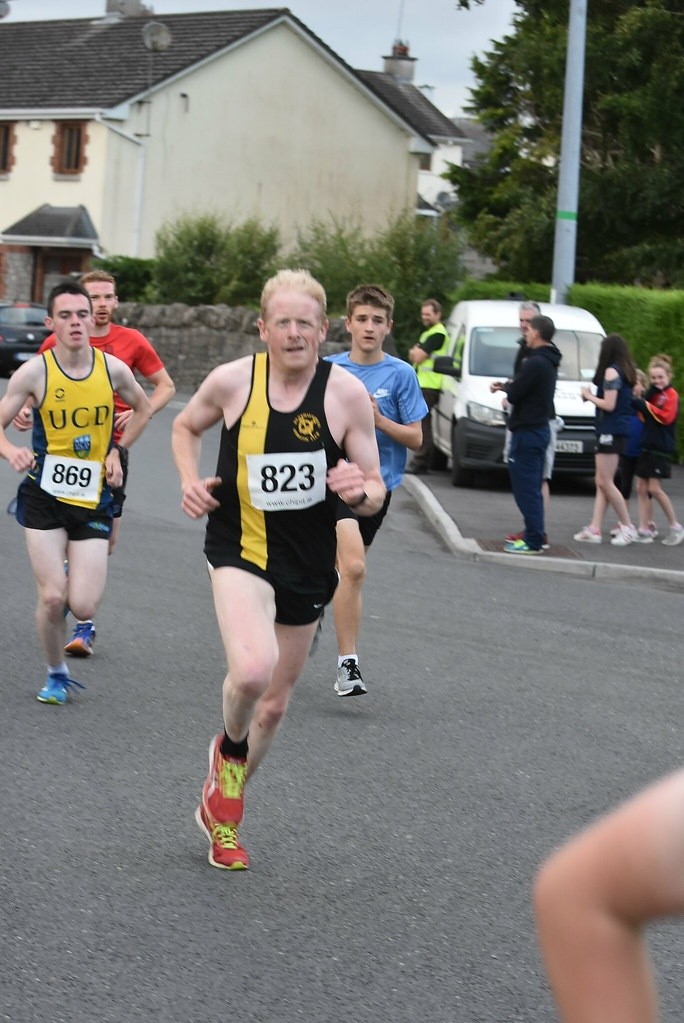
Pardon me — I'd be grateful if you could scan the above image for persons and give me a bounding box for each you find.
[307,284,429,698]
[13,270,176,657]
[574,333,684,547]
[170,269,386,869]
[0,282,154,706]
[535,770,684,1023]
[489,301,563,554]
[404,299,452,474]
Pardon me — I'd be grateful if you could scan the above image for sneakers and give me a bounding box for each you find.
[632,530,653,544]
[37,672,86,705]
[334,659,368,697]
[503,539,544,555]
[505,531,549,549]
[65,620,96,656]
[203,734,248,828]
[195,805,249,871]
[647,522,658,539]
[661,524,684,546]
[610,524,621,536]
[574,526,601,543]
[309,613,324,655]
[610,523,638,546]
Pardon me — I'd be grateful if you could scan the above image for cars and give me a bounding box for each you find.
[0,296,55,378]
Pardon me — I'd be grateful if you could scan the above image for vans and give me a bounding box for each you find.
[430,297,636,500]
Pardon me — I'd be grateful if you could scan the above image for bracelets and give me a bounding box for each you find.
[114,443,124,457]
[338,490,369,508]
